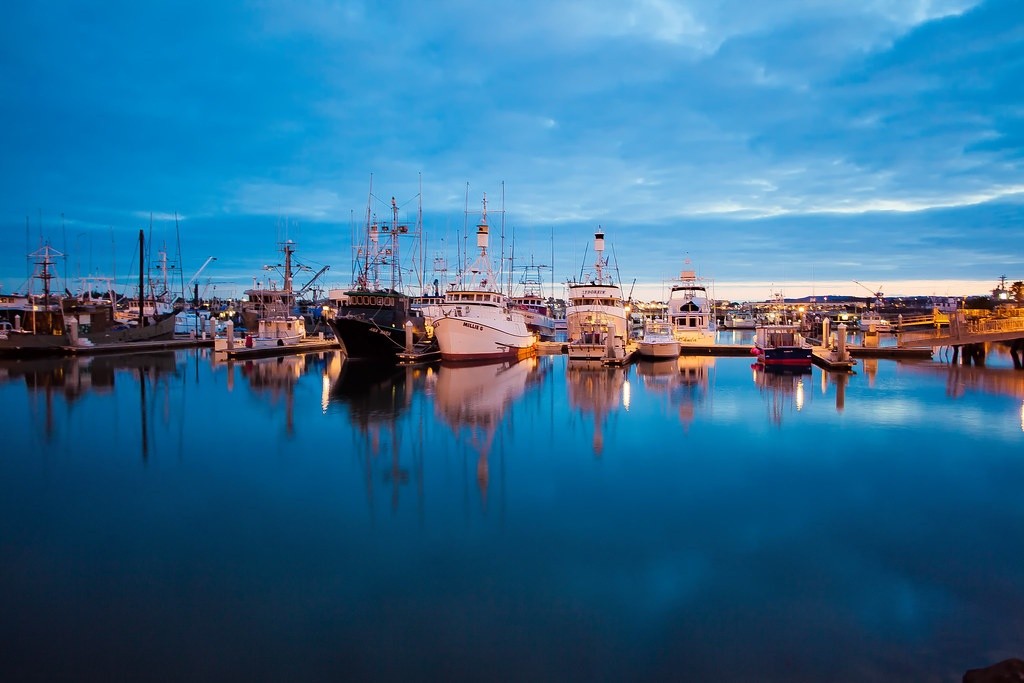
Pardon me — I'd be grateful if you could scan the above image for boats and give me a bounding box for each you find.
[565,224,639,362]
[636,321,681,359]
[723,312,755,329]
[0,170,460,364]
[661,251,717,347]
[755,284,891,332]
[429,181,542,362]
[511,227,557,342]
[749,289,812,368]
